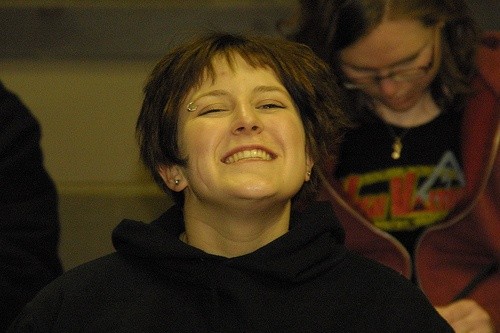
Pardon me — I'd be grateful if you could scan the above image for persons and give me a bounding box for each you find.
[1,75,67,333]
[274,0,500,333]
[7,18,458,333]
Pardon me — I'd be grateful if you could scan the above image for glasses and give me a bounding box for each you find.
[340,31,437,90]
